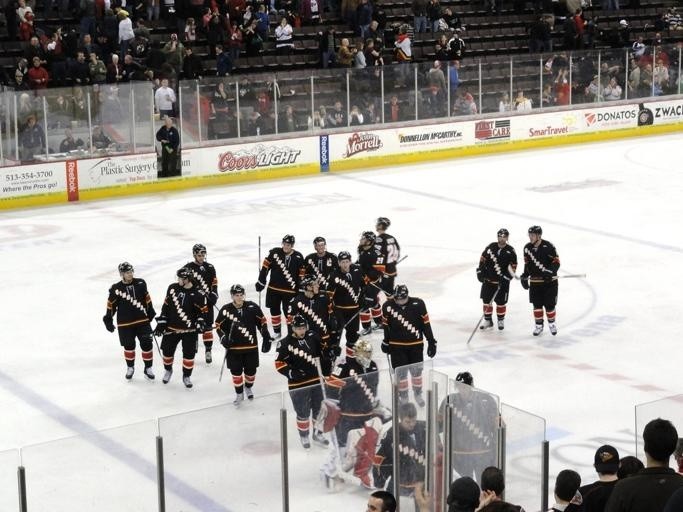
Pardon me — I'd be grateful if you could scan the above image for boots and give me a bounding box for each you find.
[232,393,244,406]
[297,428,331,449]
[143,366,155,380]
[271,333,281,338]
[204,351,212,364]
[361,322,386,335]
[479,318,494,329]
[532,324,544,337]
[245,386,255,400]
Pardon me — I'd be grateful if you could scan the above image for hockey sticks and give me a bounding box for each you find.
[507,265,586,281]
[314,358,362,486]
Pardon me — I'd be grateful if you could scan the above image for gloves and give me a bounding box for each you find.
[290,367,306,381]
[380,339,390,354]
[196,318,207,333]
[220,335,233,349]
[101,315,116,333]
[254,280,266,293]
[261,335,275,353]
[519,273,530,290]
[426,341,437,358]
[153,317,166,337]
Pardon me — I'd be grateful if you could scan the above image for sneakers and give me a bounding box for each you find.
[182,374,193,388]
[497,318,505,331]
[401,390,428,409]
[162,368,173,384]
[548,323,557,335]
[125,366,135,379]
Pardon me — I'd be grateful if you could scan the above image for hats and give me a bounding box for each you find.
[447,475,482,510]
[593,444,620,475]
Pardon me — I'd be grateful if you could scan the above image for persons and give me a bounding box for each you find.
[364,490,398,511]
[369,403,444,501]
[155,118,178,176]
[478,463,523,510]
[436,371,502,483]
[213,285,273,406]
[101,260,157,381]
[518,225,560,336]
[474,229,518,333]
[547,417,683,511]
[444,476,497,511]
[148,267,204,389]
[179,244,218,365]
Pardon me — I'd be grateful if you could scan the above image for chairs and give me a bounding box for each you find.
[0,1,71,70]
[147,24,170,48]
[190,4,364,140]
[569,2,682,69]
[378,0,567,121]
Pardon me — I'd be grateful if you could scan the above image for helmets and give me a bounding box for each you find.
[526,225,543,245]
[192,243,208,265]
[352,339,373,369]
[175,268,194,287]
[453,371,474,391]
[278,216,395,292]
[289,315,307,340]
[496,228,510,246]
[393,283,410,305]
[118,261,135,281]
[229,284,246,302]
